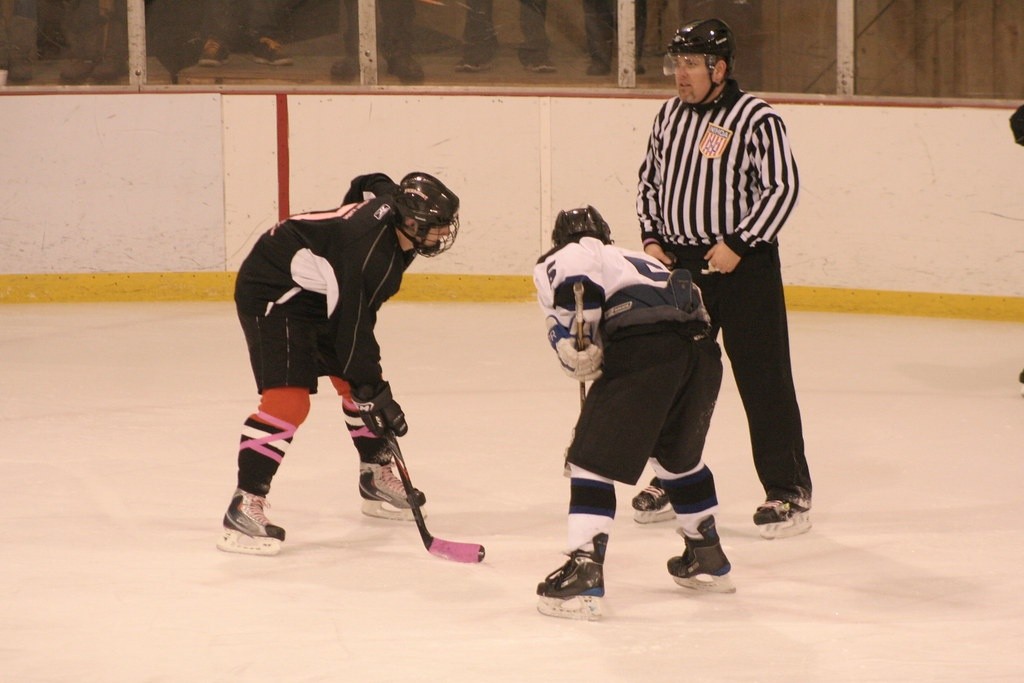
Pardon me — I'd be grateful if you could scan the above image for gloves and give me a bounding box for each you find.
[350,381,408,437]
[546,314,604,381]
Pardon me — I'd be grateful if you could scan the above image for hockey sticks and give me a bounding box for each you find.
[385,429,485,566]
[572,284,588,409]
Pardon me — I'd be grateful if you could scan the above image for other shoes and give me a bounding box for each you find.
[331,54,361,81]
[388,54,422,83]
[587,58,610,75]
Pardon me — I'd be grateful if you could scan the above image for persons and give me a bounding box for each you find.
[455,0,558,74]
[331,0,424,84]
[216,171,460,556]
[534,205,736,620]
[60,0,152,84]
[199,0,293,68]
[632,16,812,540]
[583,0,647,75]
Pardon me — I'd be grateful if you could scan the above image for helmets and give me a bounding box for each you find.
[392,172,459,258]
[667,19,734,72]
[552,205,610,246]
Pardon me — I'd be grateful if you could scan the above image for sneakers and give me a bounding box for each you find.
[667,515,737,594]
[252,37,293,65]
[216,488,285,555]
[358,462,428,521]
[198,36,232,66]
[525,55,556,72]
[753,486,812,538]
[537,533,608,621]
[455,48,490,72]
[631,486,676,525]
